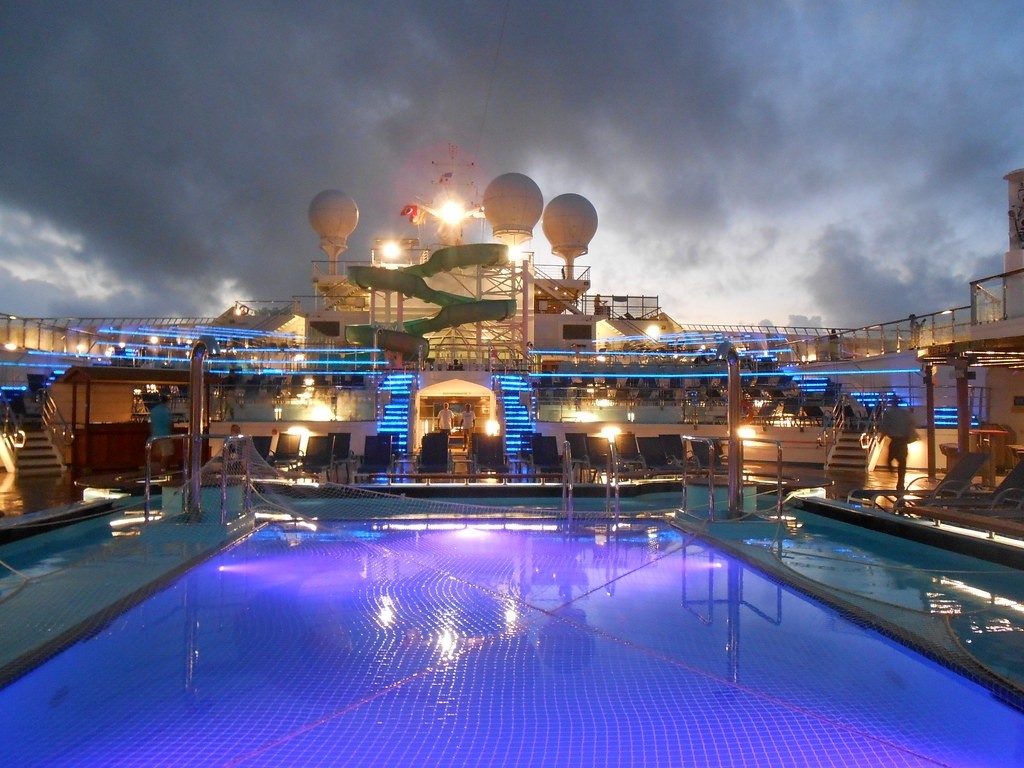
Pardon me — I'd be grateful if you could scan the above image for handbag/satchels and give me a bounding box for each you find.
[903,410,921,444]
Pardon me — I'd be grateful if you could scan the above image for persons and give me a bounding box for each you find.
[561,266,565,278]
[595,295,602,315]
[908,315,923,350]
[686,450,701,478]
[459,404,476,451]
[573,290,579,308]
[150,396,176,472]
[437,403,453,453]
[223,425,246,475]
[828,329,840,360]
[879,400,917,487]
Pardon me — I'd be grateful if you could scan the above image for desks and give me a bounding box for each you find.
[969,430,1008,450]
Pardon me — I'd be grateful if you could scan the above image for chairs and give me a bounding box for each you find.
[516,429,728,483]
[250,431,513,484]
[904,458,1024,524]
[847,452,990,508]
[540,371,883,431]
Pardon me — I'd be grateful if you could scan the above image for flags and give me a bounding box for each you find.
[400,204,418,217]
